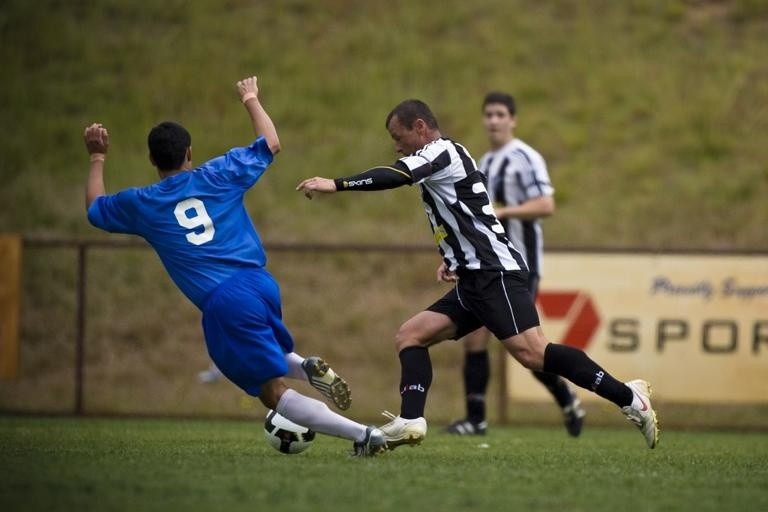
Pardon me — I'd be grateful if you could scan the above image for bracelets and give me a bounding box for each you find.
[241,91,257,104]
[89,153,105,162]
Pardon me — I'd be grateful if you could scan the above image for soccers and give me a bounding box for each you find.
[264,408,316,453]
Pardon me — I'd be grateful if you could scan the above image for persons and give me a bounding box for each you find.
[436,93,585,438]
[294,98,659,449]
[83,76,388,457]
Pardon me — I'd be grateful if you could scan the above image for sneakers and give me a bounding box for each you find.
[439,420,487,437]
[618,380,659,449]
[302,356,351,410]
[353,426,387,459]
[377,411,427,450]
[561,391,585,437]
